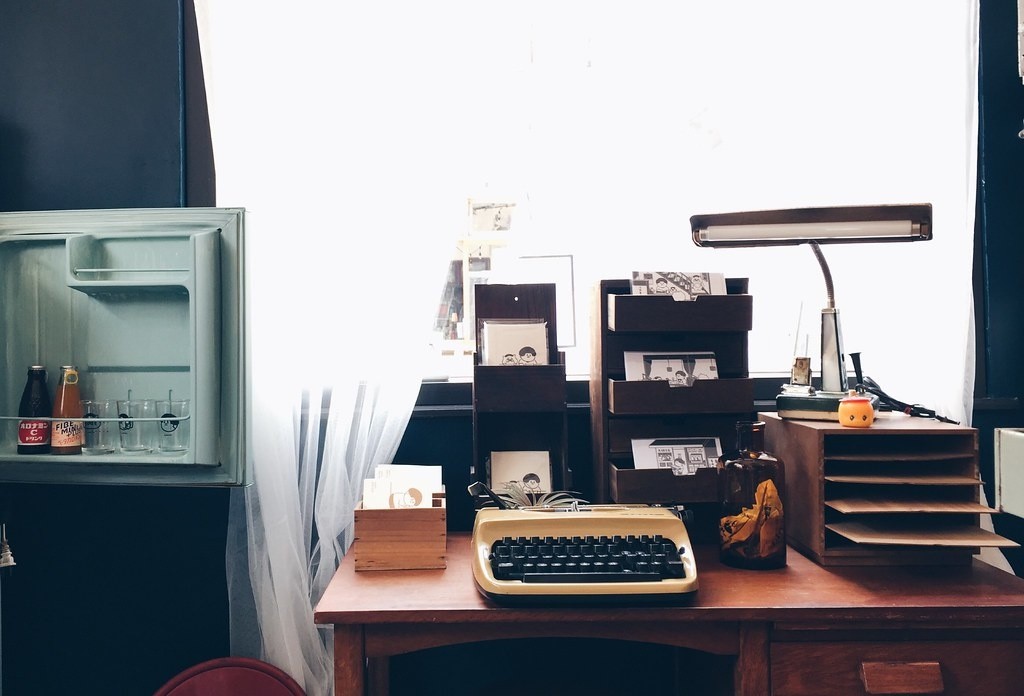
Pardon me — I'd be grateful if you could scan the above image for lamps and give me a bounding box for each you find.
[690,204,932,421]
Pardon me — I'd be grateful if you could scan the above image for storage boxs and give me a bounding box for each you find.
[353,484,446,572]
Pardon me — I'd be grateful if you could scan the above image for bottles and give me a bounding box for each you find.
[716,422,787,571]
[51,365,84,455]
[17,365,52,455]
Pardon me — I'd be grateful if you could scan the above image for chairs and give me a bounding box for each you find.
[154,657,308,696]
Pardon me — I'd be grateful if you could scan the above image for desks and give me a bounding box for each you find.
[314,533,1024,696]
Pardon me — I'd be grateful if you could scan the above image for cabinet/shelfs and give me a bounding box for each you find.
[591,277,754,546]
[758,413,1022,569]
[473,283,573,516]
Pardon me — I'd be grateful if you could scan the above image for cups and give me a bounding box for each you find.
[155,398,191,455]
[117,399,155,455]
[78,399,116,456]
